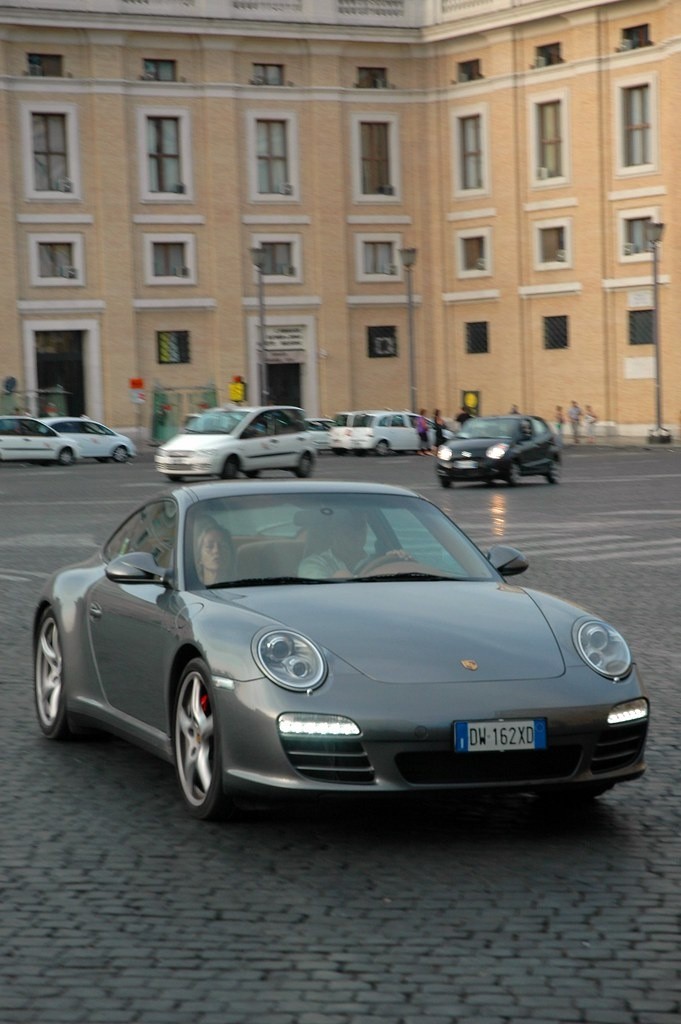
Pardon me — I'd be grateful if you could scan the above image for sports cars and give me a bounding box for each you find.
[32,477,652,820]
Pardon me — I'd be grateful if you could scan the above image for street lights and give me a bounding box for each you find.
[397,247,419,412]
[643,220,672,442]
[248,247,271,405]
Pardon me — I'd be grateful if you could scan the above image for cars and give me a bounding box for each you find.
[328,411,368,456]
[0,416,83,466]
[153,405,316,482]
[37,417,137,463]
[350,410,457,456]
[435,414,562,488]
[305,416,338,451]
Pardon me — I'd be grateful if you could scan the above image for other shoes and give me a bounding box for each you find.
[416,451,424,455]
[425,451,433,455]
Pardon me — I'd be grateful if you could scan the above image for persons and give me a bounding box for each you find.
[297,514,412,579]
[509,404,521,415]
[196,525,236,584]
[416,408,433,457]
[553,406,565,436]
[568,399,584,444]
[247,417,266,435]
[455,406,474,426]
[431,409,445,455]
[584,404,598,443]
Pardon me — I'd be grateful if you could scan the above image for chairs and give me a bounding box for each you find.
[191,515,217,583]
[236,539,303,579]
